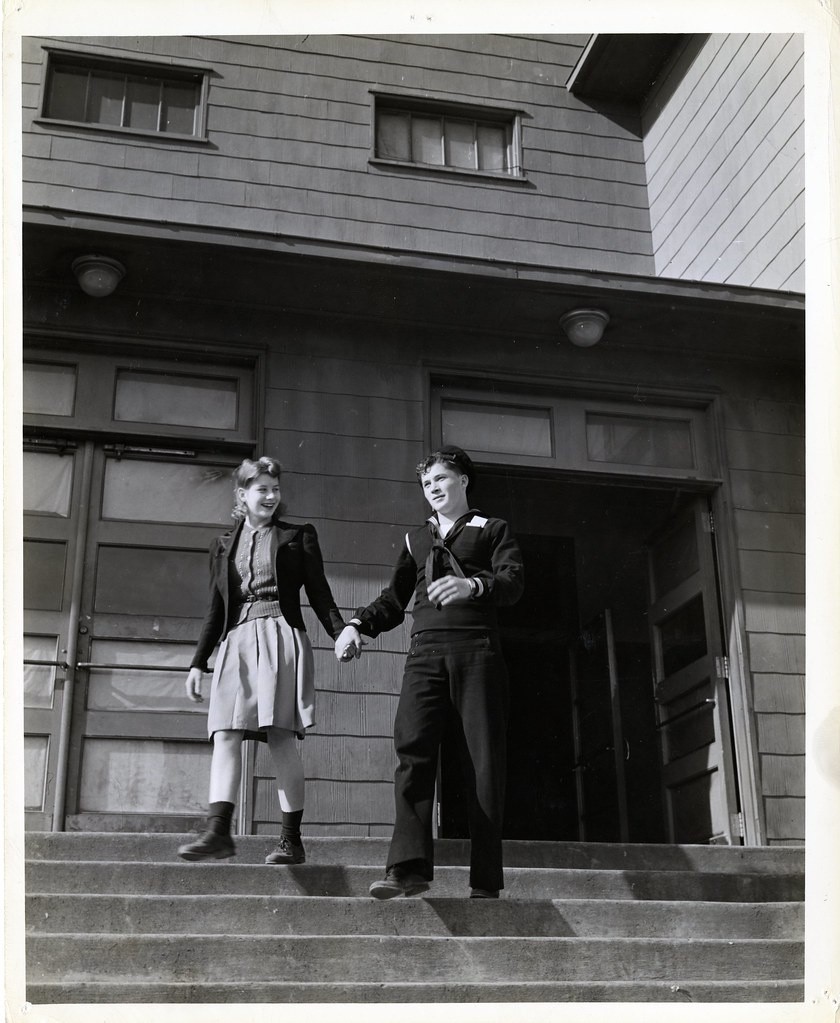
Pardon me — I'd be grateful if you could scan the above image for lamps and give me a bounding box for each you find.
[559,308,610,347]
[71,254,127,297]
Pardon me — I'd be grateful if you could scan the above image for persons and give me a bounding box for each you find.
[178,458,369,865]
[333,448,524,901]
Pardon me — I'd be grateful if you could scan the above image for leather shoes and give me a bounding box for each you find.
[369,865,431,899]
[472,889,499,900]
[178,831,237,862]
[266,839,306,865]
